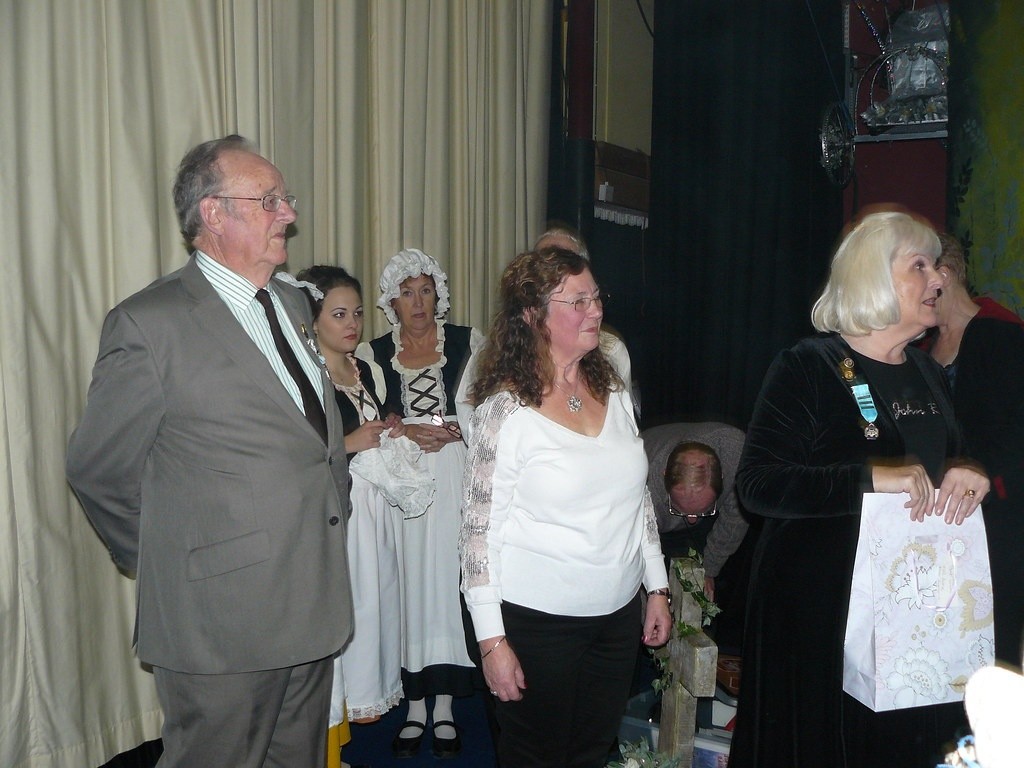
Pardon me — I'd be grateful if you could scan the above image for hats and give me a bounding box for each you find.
[376,248,450,324]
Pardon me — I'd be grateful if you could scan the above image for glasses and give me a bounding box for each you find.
[551,293,612,313]
[209,194,297,212]
[668,493,717,517]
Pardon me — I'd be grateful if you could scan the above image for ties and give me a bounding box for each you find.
[255,288,328,449]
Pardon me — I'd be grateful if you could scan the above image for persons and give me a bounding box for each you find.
[460,249,673,767]
[70,136,352,768]
[916,232,1024,671]
[328,651,351,768]
[297,266,407,724]
[354,248,488,758]
[454,227,630,447]
[727,212,990,768]
[641,421,751,625]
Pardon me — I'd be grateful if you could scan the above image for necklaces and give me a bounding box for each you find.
[555,383,582,412]
[341,378,344,384]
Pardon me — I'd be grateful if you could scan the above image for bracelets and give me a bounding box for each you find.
[481,637,505,659]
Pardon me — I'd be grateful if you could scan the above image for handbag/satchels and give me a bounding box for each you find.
[843,488,995,711]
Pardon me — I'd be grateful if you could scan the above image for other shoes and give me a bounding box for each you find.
[392,713,429,758]
[432,711,461,759]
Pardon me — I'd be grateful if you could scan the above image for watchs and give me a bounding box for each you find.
[647,590,671,603]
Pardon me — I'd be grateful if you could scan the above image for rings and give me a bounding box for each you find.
[965,490,975,499]
[430,431,432,435]
[490,690,497,696]
